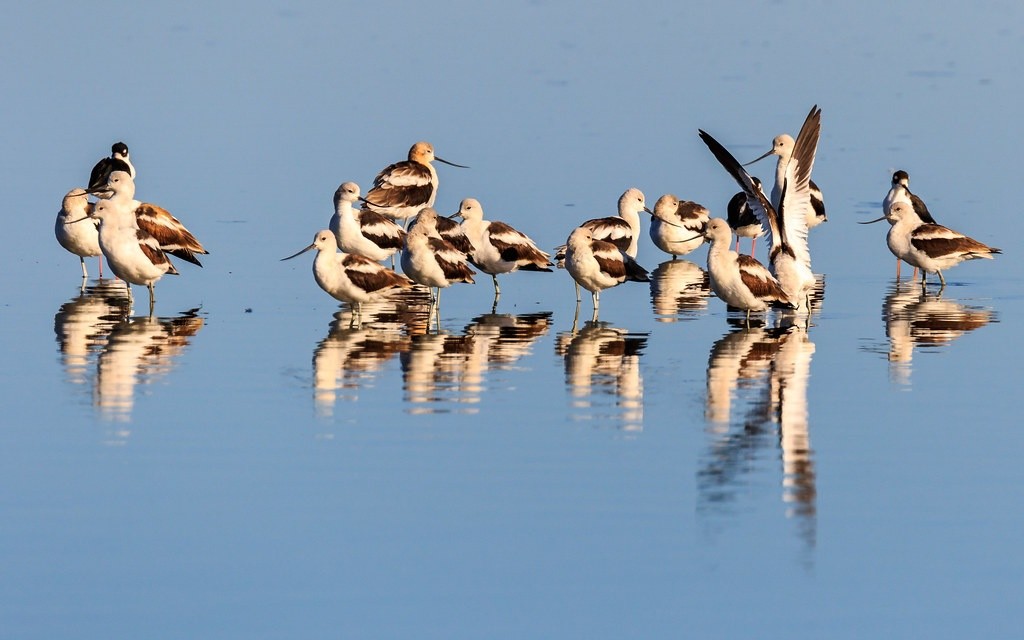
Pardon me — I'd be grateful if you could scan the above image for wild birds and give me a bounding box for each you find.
[329,183,407,262]
[551,187,683,302]
[406,208,476,261]
[726,177,765,258]
[853,280,1001,361]
[857,201,1004,297]
[312,261,820,548]
[882,170,937,280]
[279,230,415,328]
[566,227,651,323]
[359,142,471,266]
[650,194,712,259]
[64,199,180,323]
[51,275,204,420]
[399,224,478,306]
[447,199,556,294]
[737,252,801,311]
[668,218,770,319]
[697,104,822,315]
[66,171,211,268]
[85,141,136,199]
[55,189,104,277]
